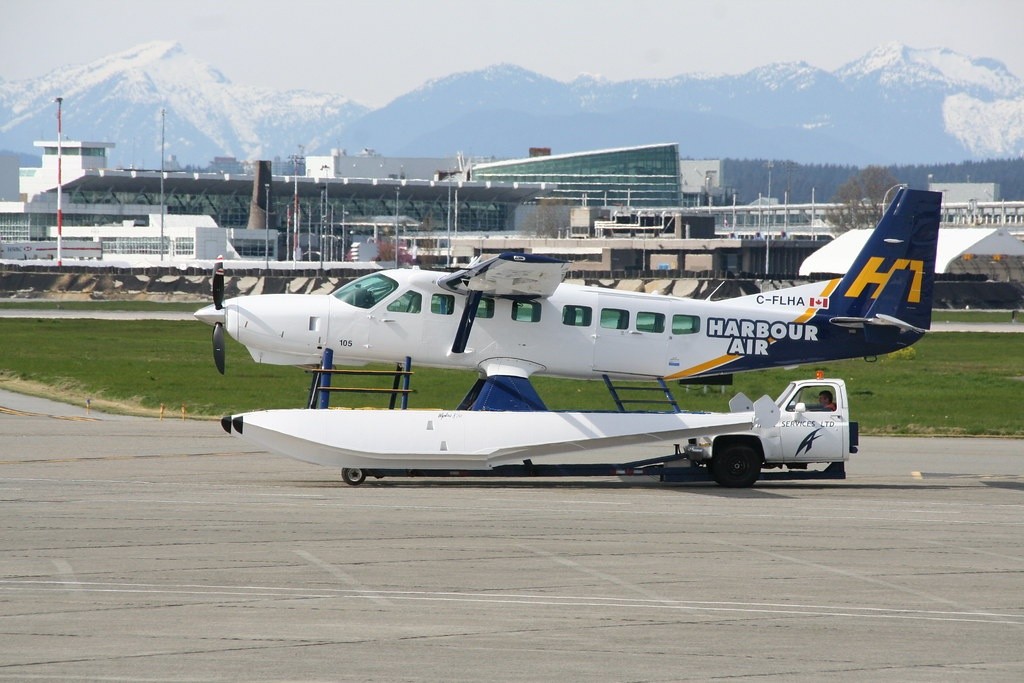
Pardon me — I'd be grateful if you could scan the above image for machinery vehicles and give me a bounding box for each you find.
[220,371,859,487]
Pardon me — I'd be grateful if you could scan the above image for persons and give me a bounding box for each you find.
[813,390,837,412]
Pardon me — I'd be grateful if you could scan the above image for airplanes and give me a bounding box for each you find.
[193,186,943,411]
